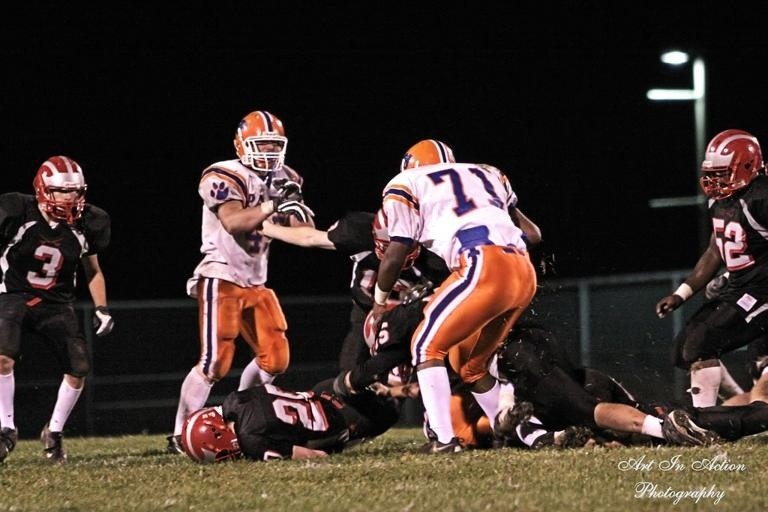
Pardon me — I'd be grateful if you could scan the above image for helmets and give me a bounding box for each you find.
[363,300,397,348]
[233,111,287,171]
[33,156,87,224]
[182,408,241,463]
[372,209,421,271]
[400,139,456,171]
[700,130,768,200]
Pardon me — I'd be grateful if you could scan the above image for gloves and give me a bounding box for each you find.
[272,178,315,223]
[656,295,684,319]
[93,306,115,337]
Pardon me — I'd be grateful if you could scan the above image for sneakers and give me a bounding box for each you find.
[502,402,591,449]
[419,428,463,454]
[167,435,182,454]
[41,423,67,464]
[662,409,720,446]
[0,427,18,463]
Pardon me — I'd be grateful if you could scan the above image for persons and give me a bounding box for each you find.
[164,110,768,466]
[1,154,116,465]
